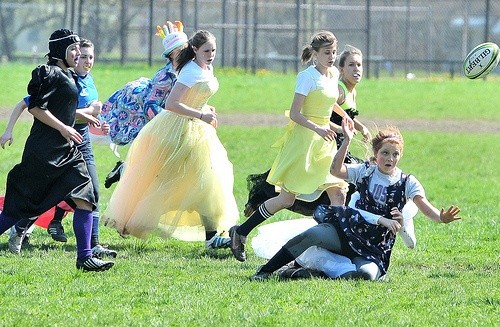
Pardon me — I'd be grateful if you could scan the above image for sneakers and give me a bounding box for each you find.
[205,233,231,251]
[104,159,125,188]
[21,236,31,248]
[90,244,117,260]
[48,220,68,242]
[8,225,23,256]
[75,258,114,273]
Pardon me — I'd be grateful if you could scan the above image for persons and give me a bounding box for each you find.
[47,118,111,242]
[104,31,216,189]
[108,30,232,252]
[243,44,372,217]
[249,116,462,282]
[280,157,421,279]
[1,29,115,271]
[0,38,118,257]
[228,31,356,261]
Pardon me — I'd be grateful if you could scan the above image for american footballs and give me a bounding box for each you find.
[461,41,500,83]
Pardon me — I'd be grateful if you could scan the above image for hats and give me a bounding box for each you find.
[155,20,188,60]
[48,28,80,62]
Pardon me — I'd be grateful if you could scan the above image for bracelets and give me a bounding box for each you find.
[200,112,203,120]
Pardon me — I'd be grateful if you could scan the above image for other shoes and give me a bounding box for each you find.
[270,265,290,277]
[228,225,246,262]
[251,264,269,283]
